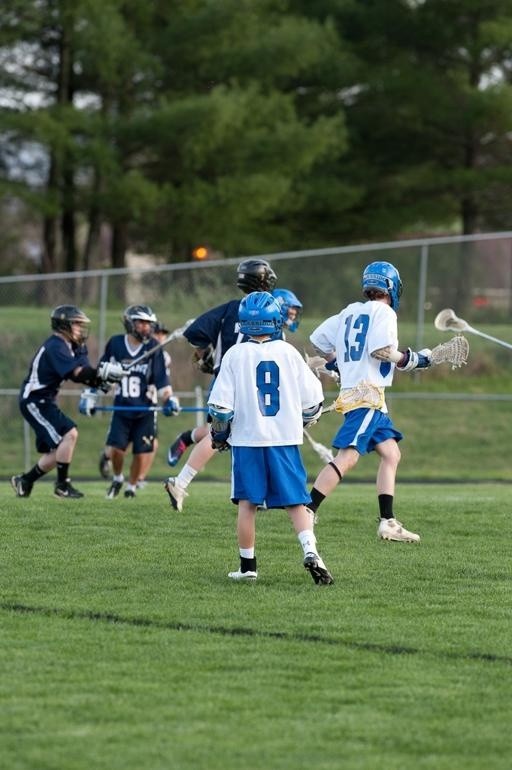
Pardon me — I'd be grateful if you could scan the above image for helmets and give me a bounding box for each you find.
[49,303,91,349]
[237,290,284,337]
[122,304,158,345]
[236,258,278,292]
[271,287,304,333]
[155,320,171,337]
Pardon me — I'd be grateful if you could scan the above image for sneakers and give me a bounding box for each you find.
[227,566,259,582]
[303,551,336,586]
[162,476,189,512]
[124,489,136,498]
[166,431,188,467]
[10,473,34,498]
[304,506,320,544]
[105,480,123,499]
[53,477,85,498]
[378,516,420,545]
[99,450,110,479]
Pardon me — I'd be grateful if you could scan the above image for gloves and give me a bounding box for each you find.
[193,346,214,376]
[78,388,97,418]
[395,346,434,371]
[96,360,131,383]
[162,395,182,417]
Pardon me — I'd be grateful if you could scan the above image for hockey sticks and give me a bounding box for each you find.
[321,382,384,415]
[302,426,335,463]
[310,355,342,384]
[431,337,469,366]
[436,307,512,350]
[125,317,197,368]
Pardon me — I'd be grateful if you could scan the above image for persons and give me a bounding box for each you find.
[162,287,304,466]
[79,307,182,496]
[167,258,280,516]
[11,306,110,499]
[213,291,333,586]
[98,321,173,490]
[303,262,433,544]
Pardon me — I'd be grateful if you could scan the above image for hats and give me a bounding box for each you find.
[360,260,403,312]
